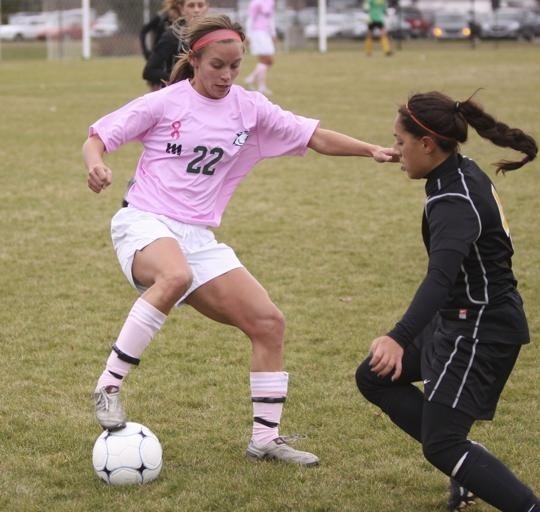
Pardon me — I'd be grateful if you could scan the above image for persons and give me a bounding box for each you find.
[362,0,397,59]
[244,0,278,97]
[354,90,540,512]
[81,12,400,468]
[140,1,174,60]
[142,1,209,92]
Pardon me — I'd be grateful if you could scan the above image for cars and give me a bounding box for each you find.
[0,8,121,40]
[298,0,540,39]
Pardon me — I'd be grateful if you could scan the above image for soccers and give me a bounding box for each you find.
[93,422,163,486]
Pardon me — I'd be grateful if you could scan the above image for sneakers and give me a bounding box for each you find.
[245,436,320,467]
[93,385,126,430]
[447,479,475,512]
